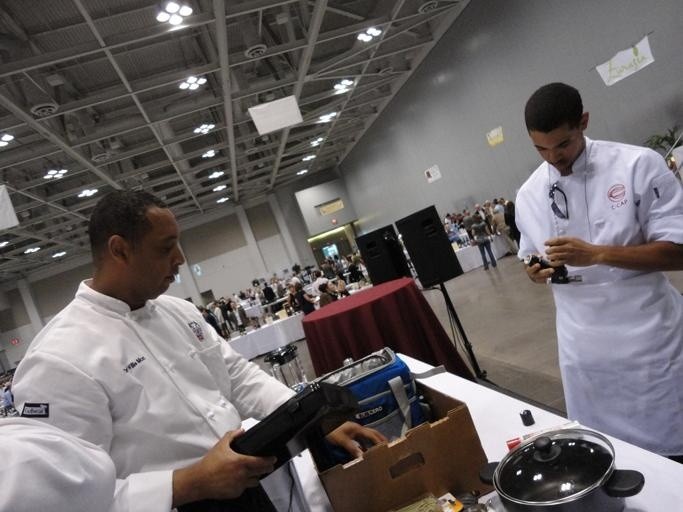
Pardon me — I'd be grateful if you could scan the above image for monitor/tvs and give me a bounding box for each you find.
[322,244,339,260]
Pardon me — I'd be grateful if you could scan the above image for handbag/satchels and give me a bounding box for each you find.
[318,346,415,429]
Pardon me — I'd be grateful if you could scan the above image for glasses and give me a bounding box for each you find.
[548,185,569,220]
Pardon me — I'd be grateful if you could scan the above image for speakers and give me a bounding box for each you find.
[355,224,413,286]
[394,204,464,289]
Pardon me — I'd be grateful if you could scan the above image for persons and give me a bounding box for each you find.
[195,271,286,341]
[286,252,366,315]
[444,195,521,271]
[10,188,387,512]
[514,82,683,462]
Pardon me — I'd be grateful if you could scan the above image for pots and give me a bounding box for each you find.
[479,428,645,512]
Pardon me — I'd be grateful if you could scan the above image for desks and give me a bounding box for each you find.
[455,233,509,273]
[302,277,477,383]
[228,284,372,360]
[241,352,683,512]
